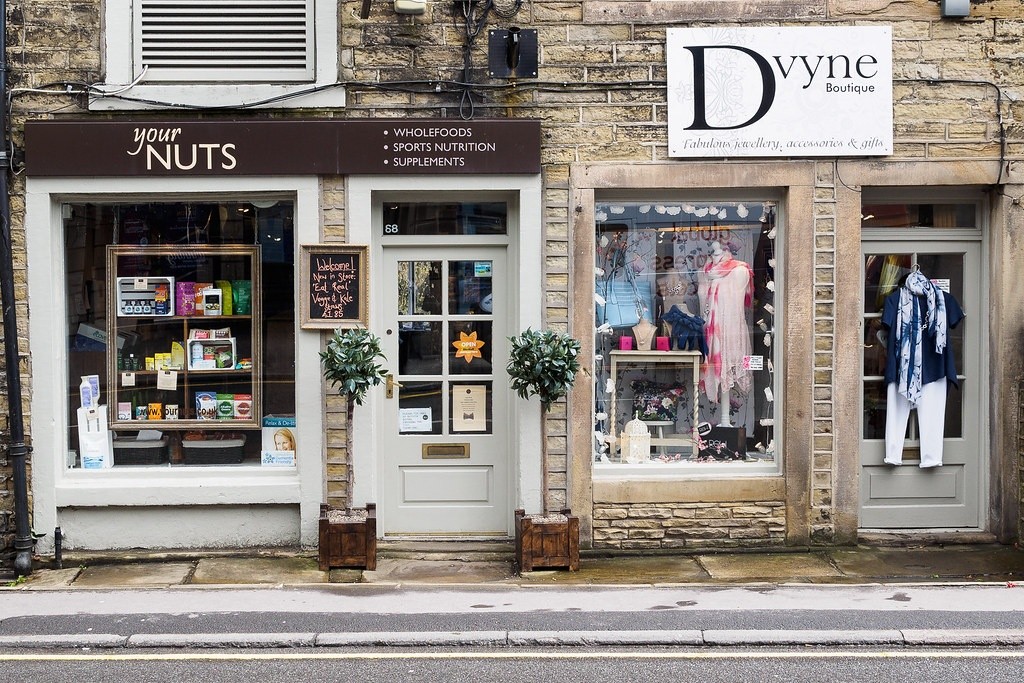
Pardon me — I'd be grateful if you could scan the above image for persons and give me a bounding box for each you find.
[273,428,296,462]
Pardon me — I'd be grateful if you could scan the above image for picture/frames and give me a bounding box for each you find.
[106,244,263,431]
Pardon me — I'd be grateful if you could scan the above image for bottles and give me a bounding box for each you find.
[129,354,135,370]
[143,300,152,314]
[125,300,133,315]
[134,300,142,315]
[168,431,182,464]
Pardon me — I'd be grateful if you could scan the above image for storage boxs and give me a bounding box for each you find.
[261,414,296,467]
[657,336,670,350]
[77,404,114,469]
[620,336,632,350]
[118,402,180,420]
[187,338,236,371]
[699,427,747,461]
[195,392,253,420]
[117,276,175,317]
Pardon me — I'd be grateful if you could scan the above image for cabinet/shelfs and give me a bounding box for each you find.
[609,347,702,459]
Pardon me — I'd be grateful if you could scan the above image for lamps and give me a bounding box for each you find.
[619,410,652,463]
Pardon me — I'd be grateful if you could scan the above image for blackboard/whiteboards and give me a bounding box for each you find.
[300,243,370,330]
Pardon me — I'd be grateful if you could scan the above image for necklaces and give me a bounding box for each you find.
[636,324,653,344]
[665,276,682,293]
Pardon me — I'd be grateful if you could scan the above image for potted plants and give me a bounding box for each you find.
[505,326,580,573]
[319,323,389,572]
